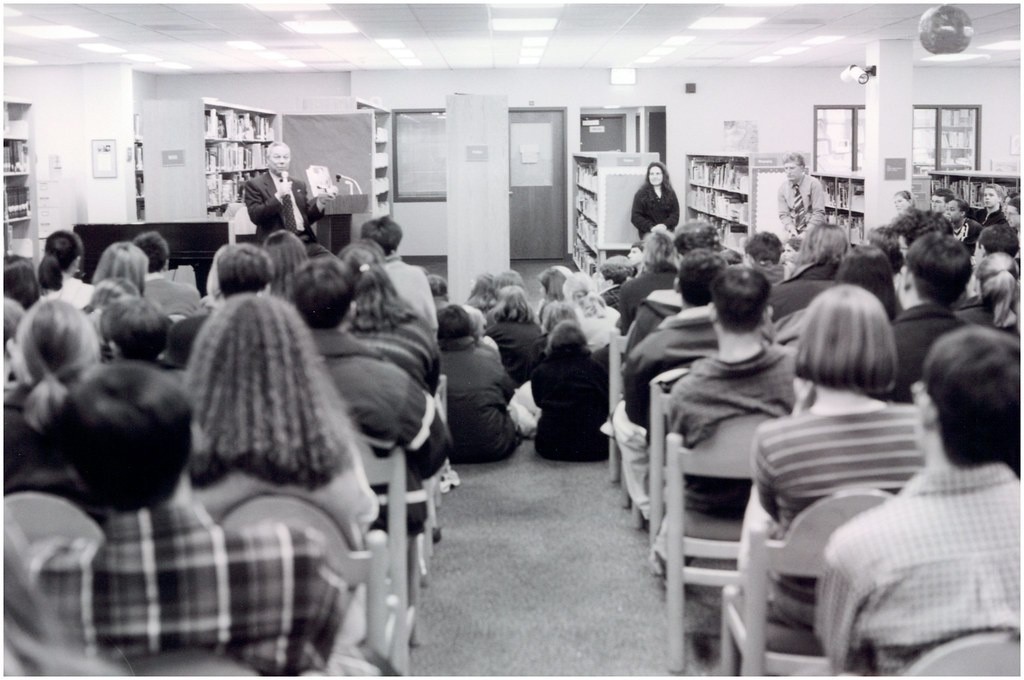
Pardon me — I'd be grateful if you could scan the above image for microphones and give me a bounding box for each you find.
[282,171,288,182]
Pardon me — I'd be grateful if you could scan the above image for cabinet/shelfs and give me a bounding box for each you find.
[573,152,660,280]
[927,170,1020,210]
[685,153,810,266]
[202,97,281,220]
[3,96,39,259]
[810,171,866,247]
[303,97,392,221]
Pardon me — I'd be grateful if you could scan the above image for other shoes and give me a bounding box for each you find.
[440,469,459,494]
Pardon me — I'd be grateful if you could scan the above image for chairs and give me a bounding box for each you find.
[608,327,1020,676]
[4,314,441,676]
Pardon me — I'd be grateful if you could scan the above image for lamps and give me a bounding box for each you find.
[840,65,876,85]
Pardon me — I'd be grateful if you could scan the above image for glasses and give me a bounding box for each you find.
[910,381,939,419]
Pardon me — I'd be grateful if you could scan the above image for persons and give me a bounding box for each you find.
[631,162,680,241]
[777,149,827,246]
[244,141,340,247]
[5,190,1019,678]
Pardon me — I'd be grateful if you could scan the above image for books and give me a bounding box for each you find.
[134,142,146,220]
[571,160,599,275]
[932,177,1020,246]
[687,156,750,256]
[204,105,276,210]
[5,139,35,219]
[822,178,863,247]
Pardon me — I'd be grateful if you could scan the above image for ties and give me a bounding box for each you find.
[279,178,297,235]
[794,184,807,234]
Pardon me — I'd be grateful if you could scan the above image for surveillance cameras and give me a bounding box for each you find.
[841,65,857,83]
[851,66,870,84]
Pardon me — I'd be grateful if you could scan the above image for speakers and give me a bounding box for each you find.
[610,68,636,85]
